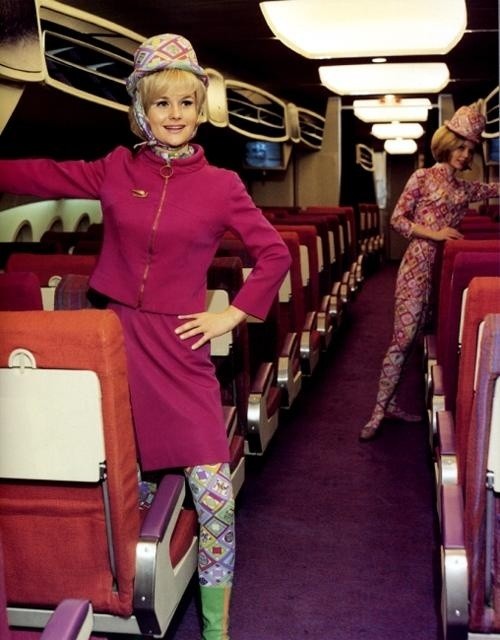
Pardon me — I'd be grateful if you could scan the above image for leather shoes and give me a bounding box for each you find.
[359,427,376,439]
[383,410,421,422]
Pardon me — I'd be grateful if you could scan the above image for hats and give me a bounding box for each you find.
[126,32,208,94]
[444,104,485,143]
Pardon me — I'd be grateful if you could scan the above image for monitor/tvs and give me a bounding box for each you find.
[482,138,500,166]
[241,129,293,171]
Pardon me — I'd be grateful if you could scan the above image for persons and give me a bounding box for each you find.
[357,97,499,440]
[3,31,295,640]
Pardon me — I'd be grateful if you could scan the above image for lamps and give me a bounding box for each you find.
[258,0,470,159]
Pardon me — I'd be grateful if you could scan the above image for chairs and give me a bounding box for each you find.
[421,205,499,638]
[0,196,384,638]
